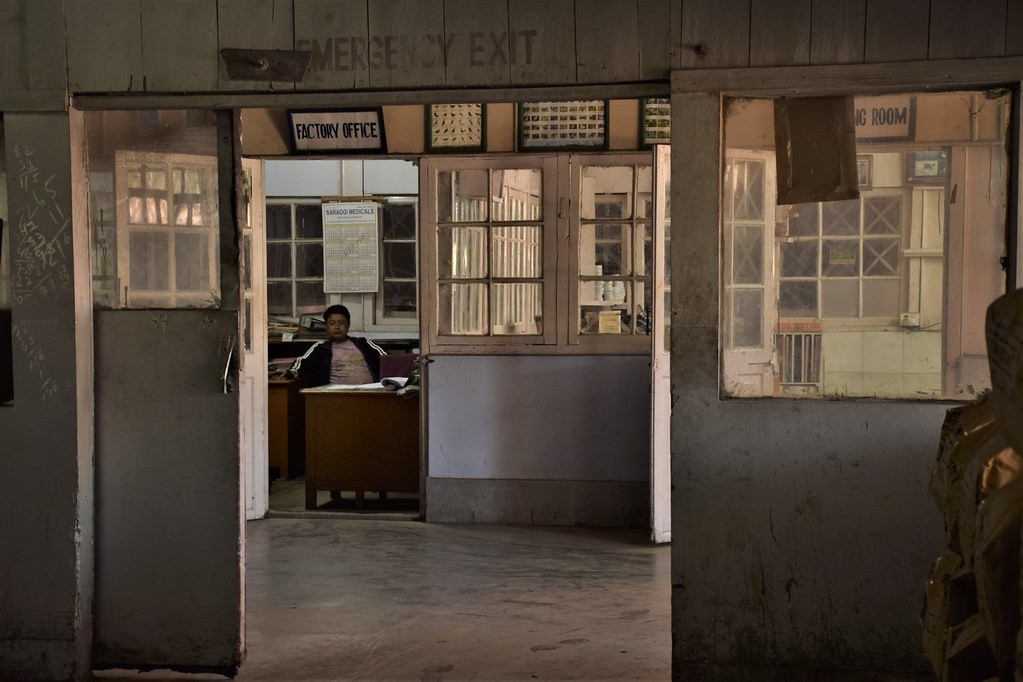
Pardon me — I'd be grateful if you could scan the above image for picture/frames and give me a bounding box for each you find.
[516,100,610,153]
[286,107,388,157]
[853,94,916,143]
[857,154,874,192]
[636,98,670,151]
[424,103,487,154]
[904,151,948,185]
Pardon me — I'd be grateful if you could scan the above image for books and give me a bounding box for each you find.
[328,377,408,390]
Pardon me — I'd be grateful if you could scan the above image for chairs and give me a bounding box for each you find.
[380,353,419,380]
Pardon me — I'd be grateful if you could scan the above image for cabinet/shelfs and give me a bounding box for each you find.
[268,370,419,510]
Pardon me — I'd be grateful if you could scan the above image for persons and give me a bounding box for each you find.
[286,305,387,385]
